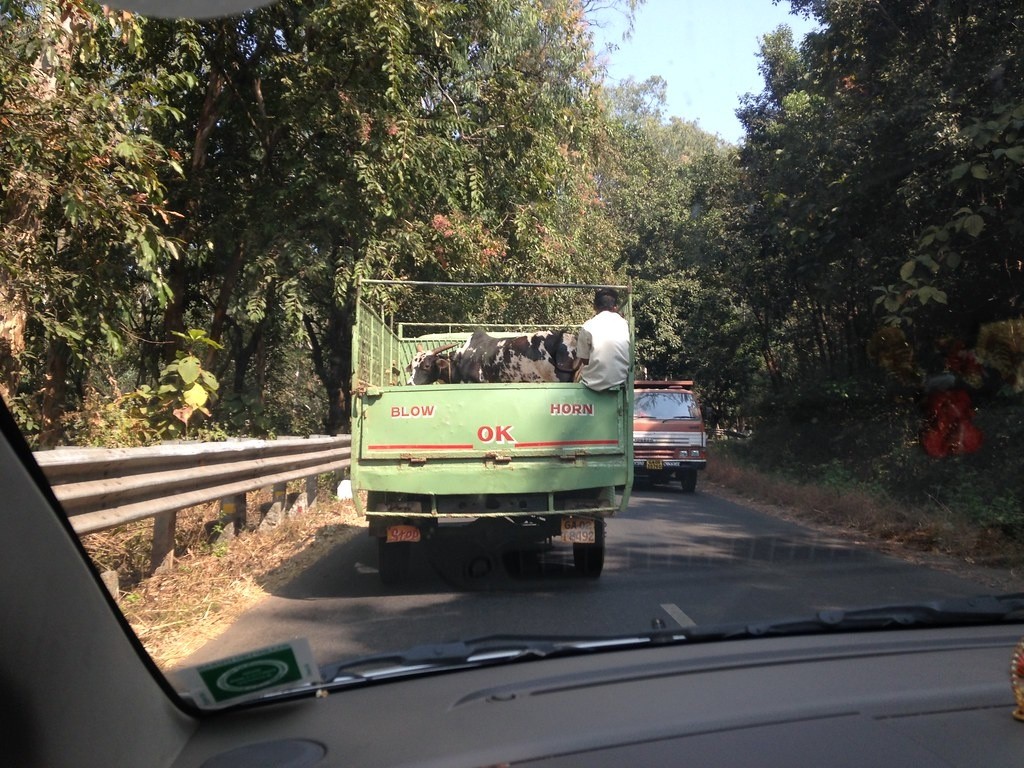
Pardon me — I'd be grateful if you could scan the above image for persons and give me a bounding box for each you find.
[572,288,630,391]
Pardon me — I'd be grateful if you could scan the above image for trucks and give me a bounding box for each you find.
[633,380,707,493]
[349,275,635,580]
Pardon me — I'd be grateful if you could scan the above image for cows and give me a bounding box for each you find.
[405,327,589,385]
